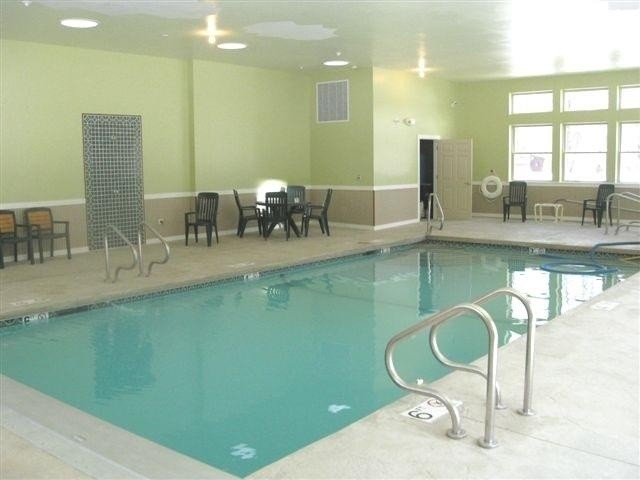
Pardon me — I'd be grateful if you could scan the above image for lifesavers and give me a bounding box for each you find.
[481,176,503,198]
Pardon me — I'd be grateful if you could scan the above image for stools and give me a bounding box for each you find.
[534,203,563,224]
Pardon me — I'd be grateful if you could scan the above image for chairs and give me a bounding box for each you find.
[503,181,527,222]
[581,184,615,226]
[233,185,332,240]
[25,207,71,263]
[184,192,219,247]
[0,210,35,269]
